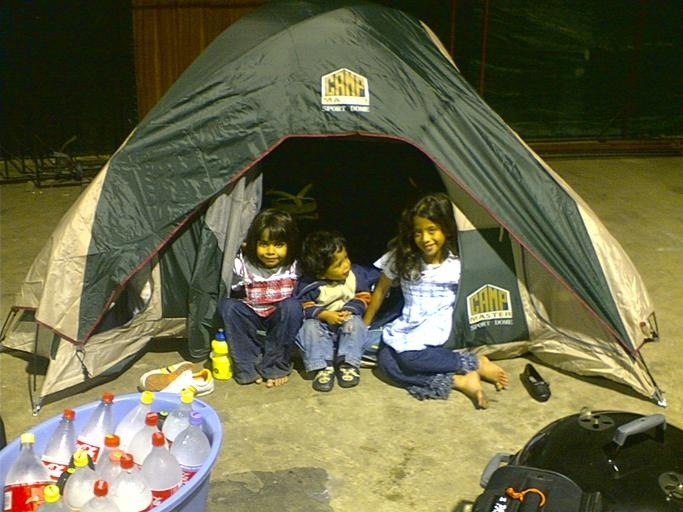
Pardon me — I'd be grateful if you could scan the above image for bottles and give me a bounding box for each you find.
[4,389,211,510]
[207,331,233,380]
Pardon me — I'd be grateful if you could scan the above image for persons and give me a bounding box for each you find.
[294,229,370,393]
[217,207,303,389]
[360,192,508,408]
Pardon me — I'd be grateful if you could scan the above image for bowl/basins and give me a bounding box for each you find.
[0,396,224,511]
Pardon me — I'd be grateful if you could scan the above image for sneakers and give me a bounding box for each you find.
[336,364,360,388]
[139,360,195,391]
[312,367,335,391]
[159,368,215,397]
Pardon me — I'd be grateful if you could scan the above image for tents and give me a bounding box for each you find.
[0,0,668,416]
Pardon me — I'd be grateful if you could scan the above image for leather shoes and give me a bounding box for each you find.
[524,364,550,401]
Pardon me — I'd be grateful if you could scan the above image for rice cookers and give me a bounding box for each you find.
[476,407,682,511]
